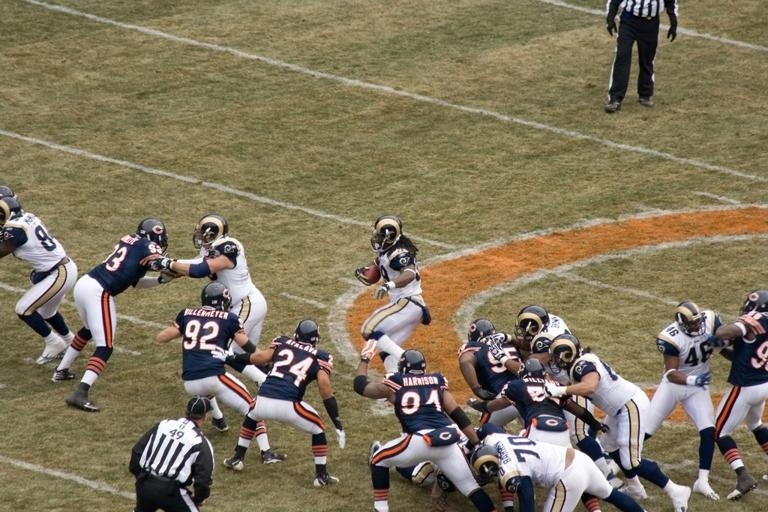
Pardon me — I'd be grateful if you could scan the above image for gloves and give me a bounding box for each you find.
[211,346,228,362]
[467,398,491,414]
[607,22,617,36]
[707,336,725,347]
[355,267,371,286]
[375,281,396,299]
[361,339,379,363]
[579,409,609,433]
[667,27,676,42]
[696,372,711,390]
[335,429,345,449]
[488,333,512,367]
[145,257,162,272]
[159,269,174,283]
[544,383,567,397]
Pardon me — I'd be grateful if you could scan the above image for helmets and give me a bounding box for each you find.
[202,282,232,311]
[517,359,547,379]
[193,213,228,249]
[136,218,168,247]
[549,334,582,370]
[514,305,548,342]
[531,333,555,354]
[436,468,457,492]
[470,446,499,478]
[0,196,21,224]
[398,349,426,375]
[468,319,496,342]
[292,320,321,347]
[0,186,18,200]
[739,289,768,316]
[675,301,707,337]
[371,216,402,253]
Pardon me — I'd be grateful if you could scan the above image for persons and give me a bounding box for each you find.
[712,290,768,501]
[367,421,503,512]
[353,338,500,512]
[355,214,431,382]
[482,306,625,491]
[542,334,694,512]
[458,317,528,431]
[0,185,79,367]
[139,212,273,389]
[51,218,215,413]
[465,358,609,511]
[463,422,649,512]
[156,281,288,463]
[129,393,215,512]
[602,0,679,113]
[643,300,734,501]
[210,318,347,488]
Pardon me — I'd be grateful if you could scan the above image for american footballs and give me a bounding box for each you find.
[363,264,381,283]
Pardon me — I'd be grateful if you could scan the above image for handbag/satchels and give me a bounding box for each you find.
[423,427,460,446]
[30,270,50,284]
[422,307,431,325]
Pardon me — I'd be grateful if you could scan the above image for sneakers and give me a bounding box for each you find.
[52,366,76,383]
[727,477,758,501]
[224,455,244,472]
[212,418,229,431]
[693,480,720,501]
[618,485,647,499]
[36,340,68,365]
[606,470,624,489]
[605,102,621,112]
[54,330,75,358]
[640,96,653,107]
[66,393,100,412]
[313,473,339,488]
[261,448,288,465]
[674,485,691,512]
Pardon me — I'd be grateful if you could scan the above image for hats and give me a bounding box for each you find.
[187,395,213,414]
